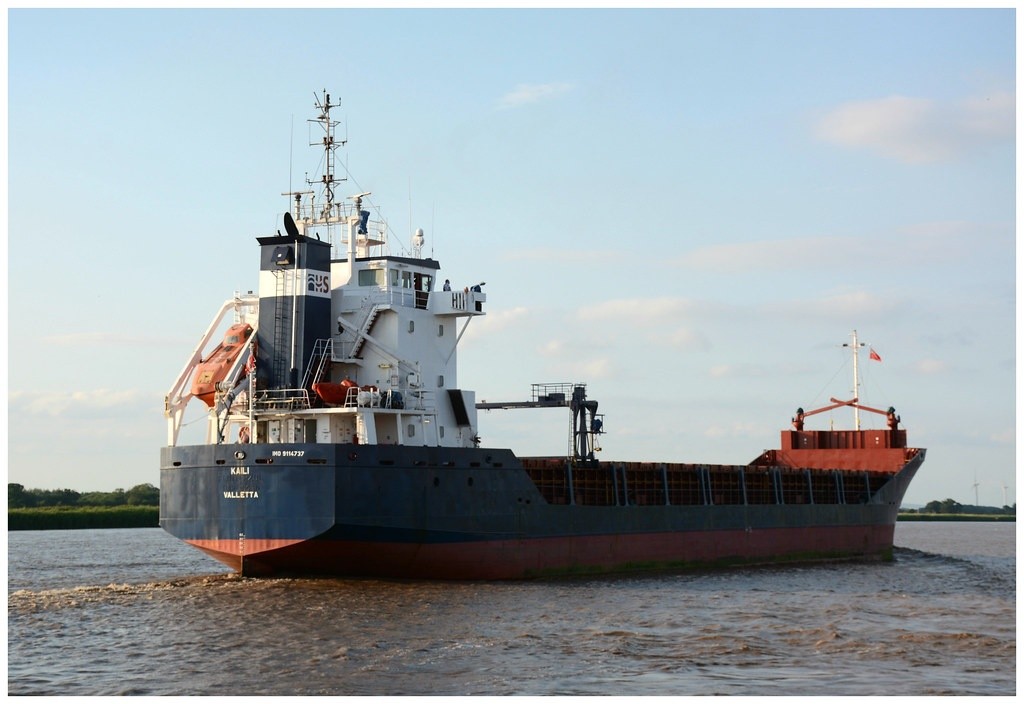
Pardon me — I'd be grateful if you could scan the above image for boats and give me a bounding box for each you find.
[149,87,930,584]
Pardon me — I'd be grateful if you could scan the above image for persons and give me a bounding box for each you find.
[443,279,451,291]
[235,431,249,459]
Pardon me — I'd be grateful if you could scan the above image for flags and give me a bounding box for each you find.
[870,348,882,361]
